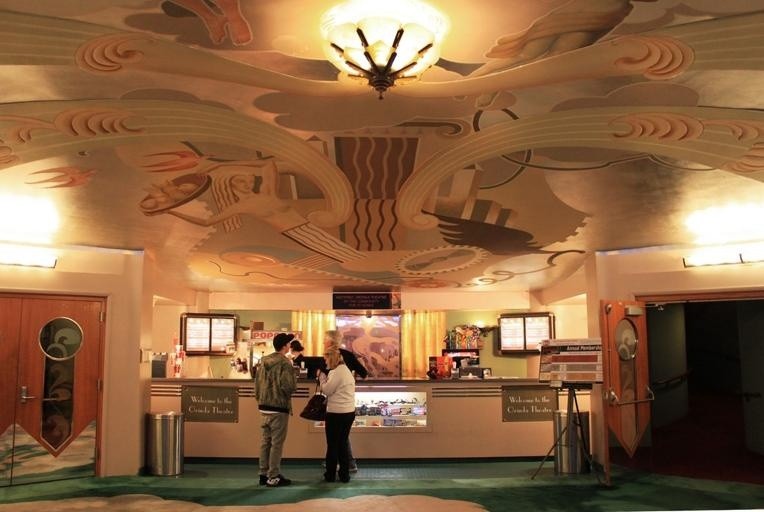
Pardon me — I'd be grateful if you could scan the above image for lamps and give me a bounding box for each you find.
[682,246,764,268]
[0,244,58,268]
[317,1,448,99]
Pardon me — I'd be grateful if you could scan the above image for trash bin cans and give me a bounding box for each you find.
[553,410,590,474]
[140,411,185,476]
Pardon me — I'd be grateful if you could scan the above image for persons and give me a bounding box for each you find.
[315,345,356,484]
[254,332,296,489]
[307,329,368,474]
[288,339,309,379]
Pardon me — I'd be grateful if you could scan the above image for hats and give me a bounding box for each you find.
[274,333,294,351]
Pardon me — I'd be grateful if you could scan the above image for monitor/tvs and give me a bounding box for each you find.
[296,356,327,377]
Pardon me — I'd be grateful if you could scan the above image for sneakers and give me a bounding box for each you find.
[260,474,291,486]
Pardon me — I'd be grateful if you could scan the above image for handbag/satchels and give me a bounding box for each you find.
[300,394,327,421]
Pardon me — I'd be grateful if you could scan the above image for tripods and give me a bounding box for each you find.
[531,389,610,488]
